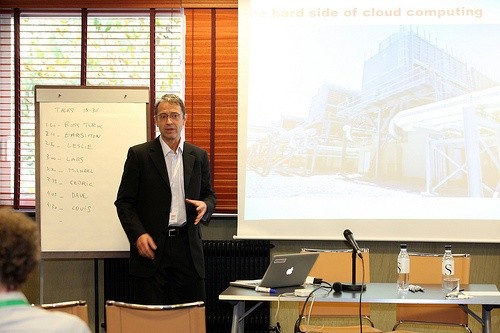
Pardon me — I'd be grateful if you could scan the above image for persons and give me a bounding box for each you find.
[0,207,93,333]
[115,95,217,305]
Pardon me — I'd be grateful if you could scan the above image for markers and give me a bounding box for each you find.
[255,286,277,293]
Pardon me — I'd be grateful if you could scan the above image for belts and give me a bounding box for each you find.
[167,226,187,237]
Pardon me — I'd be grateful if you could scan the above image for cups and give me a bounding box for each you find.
[443,277,460,299]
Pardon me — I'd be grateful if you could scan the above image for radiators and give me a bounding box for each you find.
[201,239,272,333]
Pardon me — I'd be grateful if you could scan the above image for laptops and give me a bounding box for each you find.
[229,253,320,288]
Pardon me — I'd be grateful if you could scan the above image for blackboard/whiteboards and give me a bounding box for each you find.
[33,84,150,253]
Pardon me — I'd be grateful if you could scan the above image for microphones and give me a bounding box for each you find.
[343,229,363,258]
[332,282,342,292]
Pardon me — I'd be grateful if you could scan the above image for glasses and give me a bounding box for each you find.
[156,113,183,121]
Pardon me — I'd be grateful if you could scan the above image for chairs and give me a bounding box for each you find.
[300,248,382,333]
[105,300,206,332]
[391,252,470,333]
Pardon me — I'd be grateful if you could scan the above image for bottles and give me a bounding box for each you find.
[396,244,411,291]
[441,245,455,292]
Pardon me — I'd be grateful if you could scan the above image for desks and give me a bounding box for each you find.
[219,280,500,333]
